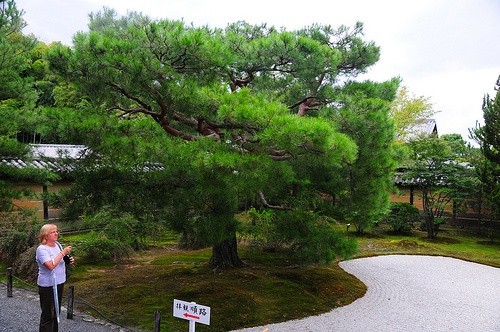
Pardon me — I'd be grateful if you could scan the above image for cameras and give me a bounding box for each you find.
[63,254,72,264]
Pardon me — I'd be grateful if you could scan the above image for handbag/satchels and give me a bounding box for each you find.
[55,241,71,278]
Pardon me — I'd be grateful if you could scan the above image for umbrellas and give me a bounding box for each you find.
[51,258,59,324]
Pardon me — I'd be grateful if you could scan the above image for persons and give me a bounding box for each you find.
[36,224,74,332]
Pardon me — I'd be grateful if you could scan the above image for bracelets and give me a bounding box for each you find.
[60,251,65,256]
